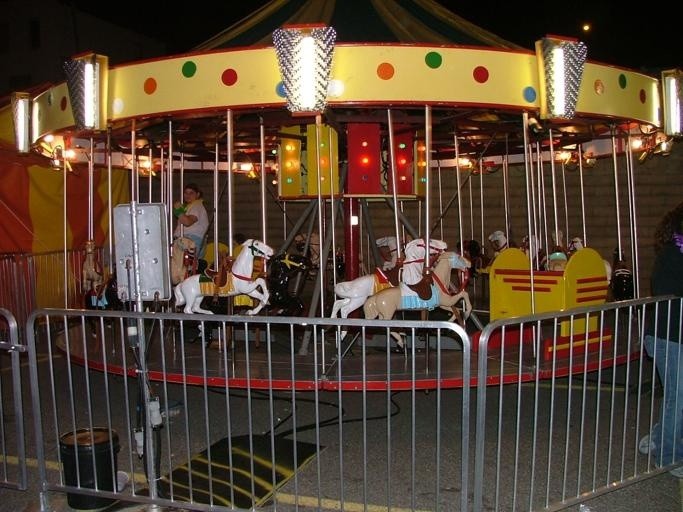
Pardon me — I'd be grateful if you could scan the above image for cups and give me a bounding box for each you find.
[116,470,129,492]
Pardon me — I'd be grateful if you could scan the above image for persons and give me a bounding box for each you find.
[635,197,683,478]
[170,182,209,262]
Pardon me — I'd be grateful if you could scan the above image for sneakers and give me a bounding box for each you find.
[637,419,683,481]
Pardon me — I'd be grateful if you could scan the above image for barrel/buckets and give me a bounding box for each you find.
[59,428,120,509]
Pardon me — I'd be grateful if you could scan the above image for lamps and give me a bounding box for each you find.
[661,69,683,137]
[559,150,597,171]
[34,135,74,173]
[62,51,108,132]
[535,35,587,123]
[11,92,33,154]
[272,21,336,116]
[633,124,674,164]
